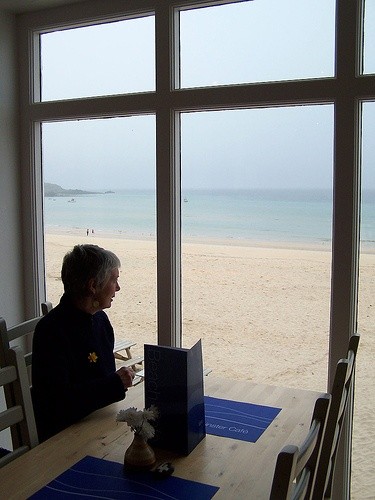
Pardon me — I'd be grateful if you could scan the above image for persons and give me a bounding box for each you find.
[30,244,137,445]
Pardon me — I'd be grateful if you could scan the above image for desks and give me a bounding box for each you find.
[0,376,325,500]
[114,339,137,373]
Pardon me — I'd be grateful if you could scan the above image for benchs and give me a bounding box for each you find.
[116,355,144,372]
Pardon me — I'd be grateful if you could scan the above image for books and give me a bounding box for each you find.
[143,338,207,457]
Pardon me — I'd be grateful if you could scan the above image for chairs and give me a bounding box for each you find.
[0,303,52,452]
[0,345,39,470]
[312,332,361,500]
[269,393,332,500]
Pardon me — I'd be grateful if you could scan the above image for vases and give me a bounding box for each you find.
[123,432,156,477]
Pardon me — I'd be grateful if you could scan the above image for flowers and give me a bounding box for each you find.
[116,405,159,442]
